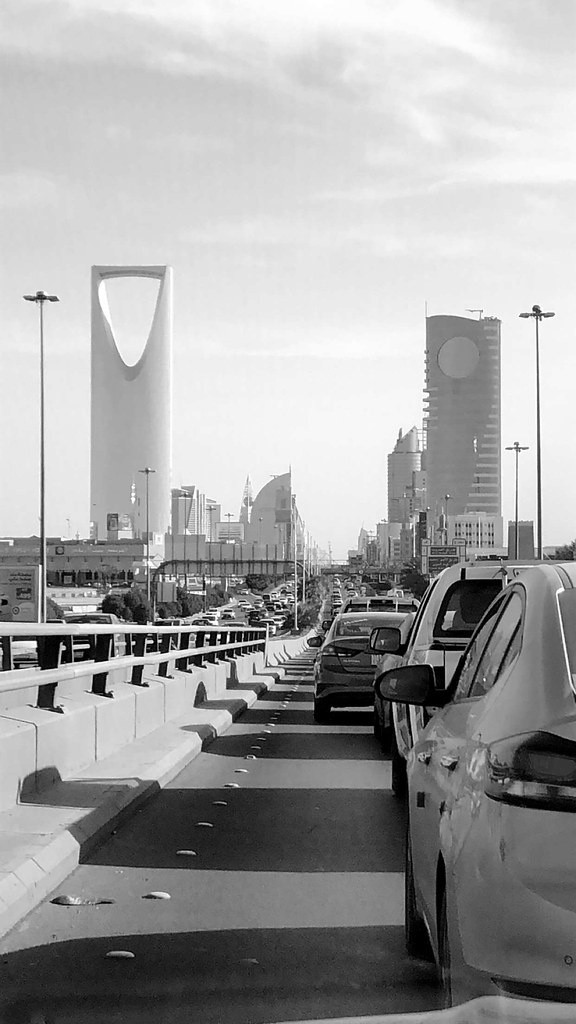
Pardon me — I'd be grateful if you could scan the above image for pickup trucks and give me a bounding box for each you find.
[369,557,576,805]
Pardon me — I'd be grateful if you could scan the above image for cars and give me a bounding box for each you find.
[376,563,576,1012]
[331,578,357,619]
[192,579,299,636]
[306,612,420,725]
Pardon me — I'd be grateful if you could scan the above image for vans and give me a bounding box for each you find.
[322,597,421,640]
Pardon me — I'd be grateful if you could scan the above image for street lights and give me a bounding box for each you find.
[180,493,192,589]
[224,513,232,569]
[23,290,60,623]
[206,506,217,585]
[138,466,155,624]
[518,305,554,560]
[505,441,529,559]
